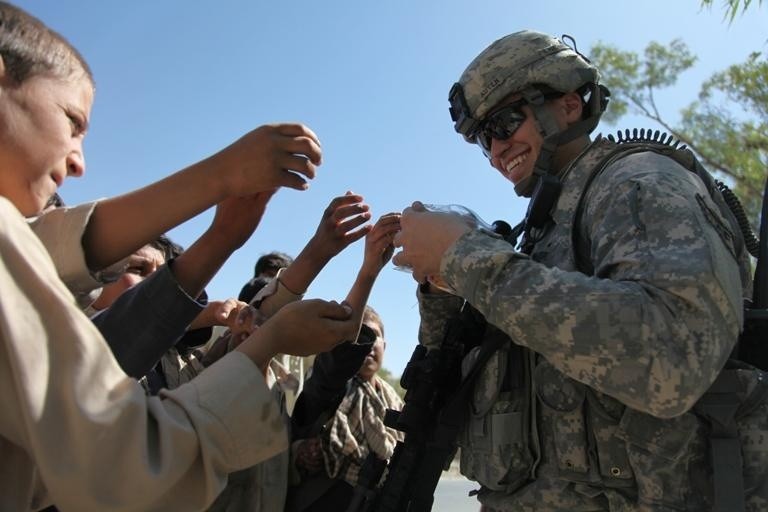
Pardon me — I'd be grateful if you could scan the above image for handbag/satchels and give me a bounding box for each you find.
[458,344,534,495]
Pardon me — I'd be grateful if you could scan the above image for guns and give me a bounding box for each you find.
[356,221,516,512]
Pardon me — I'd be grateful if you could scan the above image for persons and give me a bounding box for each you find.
[386,25,768,512]
[1,0,405,509]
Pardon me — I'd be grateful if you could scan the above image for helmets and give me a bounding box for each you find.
[448,30,603,145]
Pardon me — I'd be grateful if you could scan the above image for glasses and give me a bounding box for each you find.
[475,90,564,159]
